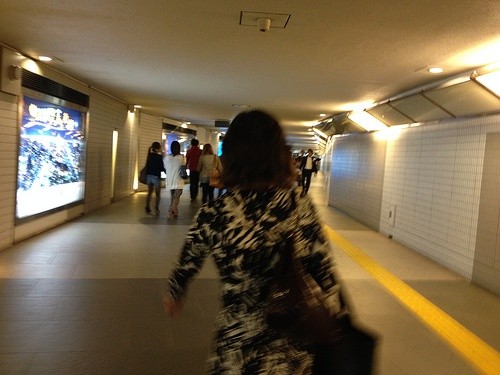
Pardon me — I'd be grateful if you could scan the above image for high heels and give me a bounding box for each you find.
[167,207,178,221]
[145,206,152,213]
[155,207,160,214]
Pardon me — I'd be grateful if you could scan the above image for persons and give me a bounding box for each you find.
[144,141,166,216]
[198,143,222,207]
[164,108,354,375]
[185,138,204,203]
[286,149,320,187]
[299,149,317,192]
[163,141,187,221]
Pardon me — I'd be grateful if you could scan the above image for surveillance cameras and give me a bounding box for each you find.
[257,19,271,33]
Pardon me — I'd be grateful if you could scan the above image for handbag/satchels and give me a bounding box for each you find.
[315,284,377,375]
[139,167,148,184]
[262,191,347,356]
[312,157,320,172]
[179,154,189,179]
[209,155,225,188]
